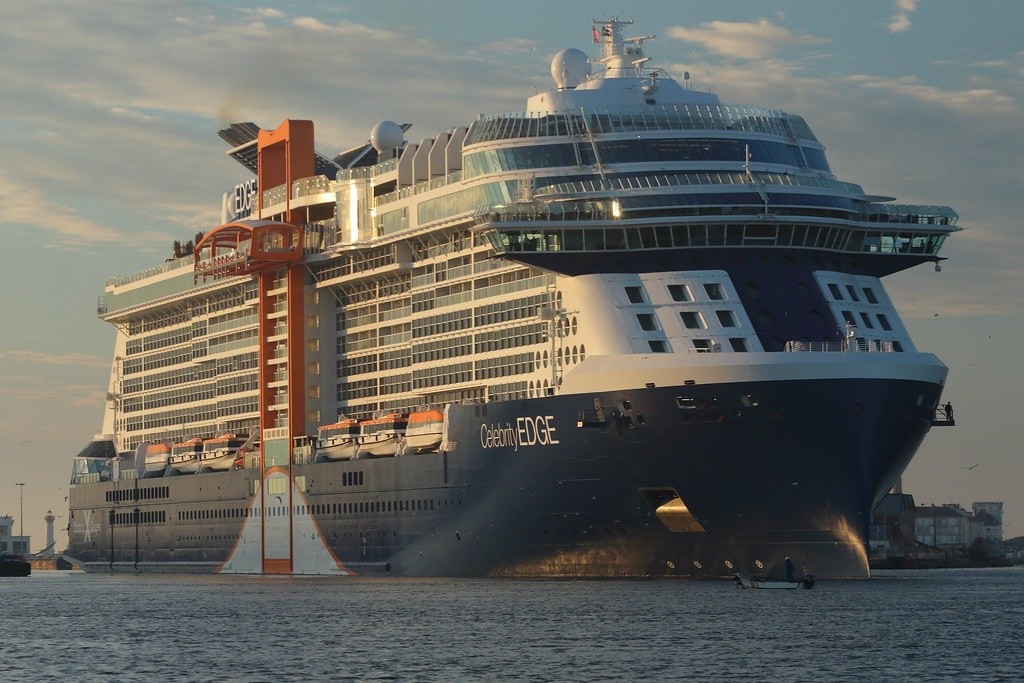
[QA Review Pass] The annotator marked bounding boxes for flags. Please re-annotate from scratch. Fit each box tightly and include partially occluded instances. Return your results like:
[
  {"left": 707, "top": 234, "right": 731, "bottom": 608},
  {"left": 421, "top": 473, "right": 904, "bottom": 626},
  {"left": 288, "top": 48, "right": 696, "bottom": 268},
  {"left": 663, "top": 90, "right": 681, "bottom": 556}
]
[
  {"left": 592, "top": 27, "right": 602, "bottom": 42},
  {"left": 601, "top": 27, "right": 611, "bottom": 37}
]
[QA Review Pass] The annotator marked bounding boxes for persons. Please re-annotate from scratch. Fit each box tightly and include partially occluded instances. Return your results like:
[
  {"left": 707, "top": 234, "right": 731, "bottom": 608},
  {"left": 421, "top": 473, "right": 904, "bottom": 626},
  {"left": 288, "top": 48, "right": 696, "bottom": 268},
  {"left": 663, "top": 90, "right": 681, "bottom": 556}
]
[
  {"left": 784, "top": 556, "right": 795, "bottom": 583},
  {"left": 920, "top": 237, "right": 934, "bottom": 254},
  {"left": 509, "top": 233, "right": 537, "bottom": 252},
  {"left": 944, "top": 401, "right": 953, "bottom": 422}
]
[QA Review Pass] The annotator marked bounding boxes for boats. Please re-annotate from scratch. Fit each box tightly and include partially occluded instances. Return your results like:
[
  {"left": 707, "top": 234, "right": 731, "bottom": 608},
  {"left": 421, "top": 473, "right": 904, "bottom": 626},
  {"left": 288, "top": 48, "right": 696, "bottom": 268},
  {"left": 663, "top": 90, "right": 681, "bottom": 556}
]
[
  {"left": 200, "top": 434, "right": 242, "bottom": 471},
  {"left": 356, "top": 413, "right": 408, "bottom": 456},
  {"left": 731, "top": 570, "right": 816, "bottom": 589},
  {"left": 145, "top": 444, "right": 173, "bottom": 474},
  {"left": 170, "top": 438, "right": 204, "bottom": 473},
  {"left": 0, "top": 554, "right": 32, "bottom": 577},
  {"left": 316, "top": 417, "right": 370, "bottom": 460},
  {"left": 405, "top": 410, "right": 444, "bottom": 449}
]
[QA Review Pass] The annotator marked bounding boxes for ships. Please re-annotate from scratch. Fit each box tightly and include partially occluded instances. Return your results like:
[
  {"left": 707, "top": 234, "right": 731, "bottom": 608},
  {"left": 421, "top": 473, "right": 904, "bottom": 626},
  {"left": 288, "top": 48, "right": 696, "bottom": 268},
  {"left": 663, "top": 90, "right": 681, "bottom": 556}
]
[{"left": 69, "top": 16, "right": 965, "bottom": 582}]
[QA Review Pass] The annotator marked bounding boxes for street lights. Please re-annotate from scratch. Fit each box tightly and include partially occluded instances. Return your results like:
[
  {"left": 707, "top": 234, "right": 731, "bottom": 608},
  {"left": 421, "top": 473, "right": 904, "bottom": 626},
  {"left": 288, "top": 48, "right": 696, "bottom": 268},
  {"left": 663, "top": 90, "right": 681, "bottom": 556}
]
[
  {"left": 921, "top": 503, "right": 926, "bottom": 544},
  {"left": 15, "top": 482, "right": 25, "bottom": 556},
  {"left": 932, "top": 502, "right": 936, "bottom": 548}
]
